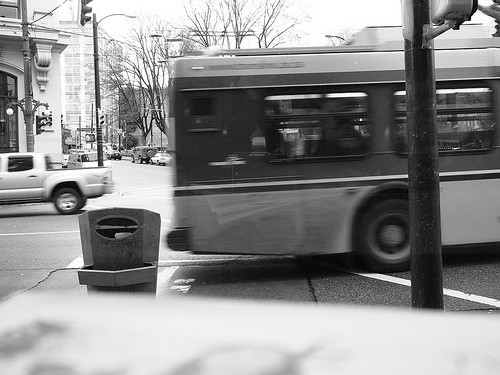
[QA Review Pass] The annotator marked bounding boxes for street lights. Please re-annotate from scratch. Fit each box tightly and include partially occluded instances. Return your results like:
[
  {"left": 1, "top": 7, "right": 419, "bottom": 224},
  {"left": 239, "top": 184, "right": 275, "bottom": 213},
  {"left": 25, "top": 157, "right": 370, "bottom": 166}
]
[{"left": 91, "top": 13, "right": 137, "bottom": 166}]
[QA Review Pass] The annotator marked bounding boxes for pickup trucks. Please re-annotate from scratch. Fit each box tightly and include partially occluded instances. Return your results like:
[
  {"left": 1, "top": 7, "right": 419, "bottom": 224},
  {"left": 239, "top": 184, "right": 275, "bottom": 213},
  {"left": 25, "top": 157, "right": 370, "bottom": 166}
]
[{"left": 1, "top": 153, "right": 113, "bottom": 215}]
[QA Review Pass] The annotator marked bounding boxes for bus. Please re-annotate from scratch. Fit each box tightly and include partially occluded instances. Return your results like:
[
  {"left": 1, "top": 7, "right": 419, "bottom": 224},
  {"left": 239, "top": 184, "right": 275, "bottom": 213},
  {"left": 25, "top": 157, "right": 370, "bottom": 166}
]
[{"left": 162, "top": 25, "right": 500, "bottom": 273}]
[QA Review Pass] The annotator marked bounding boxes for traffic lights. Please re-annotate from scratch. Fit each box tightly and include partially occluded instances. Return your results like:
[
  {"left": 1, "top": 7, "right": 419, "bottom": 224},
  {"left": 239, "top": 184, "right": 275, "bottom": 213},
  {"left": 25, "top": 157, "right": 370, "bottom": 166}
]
[
  {"left": 35, "top": 115, "right": 46, "bottom": 137},
  {"left": 76, "top": 0, "right": 92, "bottom": 33},
  {"left": 98, "top": 115, "right": 106, "bottom": 127}
]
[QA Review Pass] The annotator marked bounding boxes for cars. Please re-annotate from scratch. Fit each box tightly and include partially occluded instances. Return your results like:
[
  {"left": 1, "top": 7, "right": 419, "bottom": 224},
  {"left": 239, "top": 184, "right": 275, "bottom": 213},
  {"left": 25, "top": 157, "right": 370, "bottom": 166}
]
[{"left": 70, "top": 143, "right": 171, "bottom": 167}]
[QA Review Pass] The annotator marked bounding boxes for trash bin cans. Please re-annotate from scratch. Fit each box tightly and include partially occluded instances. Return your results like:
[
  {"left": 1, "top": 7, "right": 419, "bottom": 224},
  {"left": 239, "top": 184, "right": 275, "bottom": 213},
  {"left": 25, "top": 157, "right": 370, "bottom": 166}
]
[{"left": 76, "top": 208, "right": 161, "bottom": 299}]
